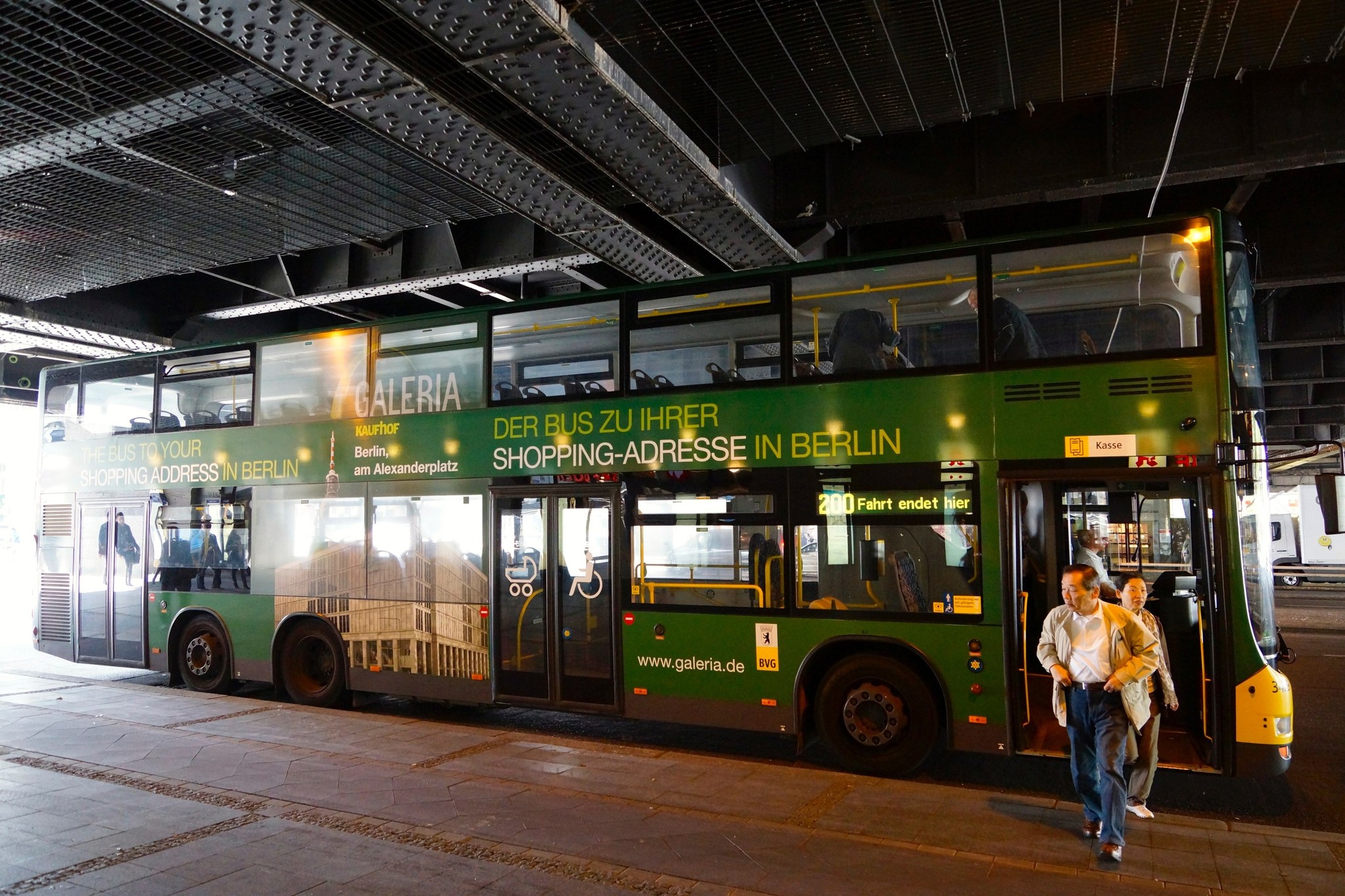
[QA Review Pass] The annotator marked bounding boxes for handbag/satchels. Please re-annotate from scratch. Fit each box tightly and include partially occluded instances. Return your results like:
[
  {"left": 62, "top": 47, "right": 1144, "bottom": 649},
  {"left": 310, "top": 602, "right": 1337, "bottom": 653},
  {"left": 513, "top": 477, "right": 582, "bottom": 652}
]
[
  {"left": 127, "top": 545, "right": 141, "bottom": 564},
  {"left": 244, "top": 566, "right": 251, "bottom": 577},
  {"left": 227, "top": 550, "right": 232, "bottom": 563}
]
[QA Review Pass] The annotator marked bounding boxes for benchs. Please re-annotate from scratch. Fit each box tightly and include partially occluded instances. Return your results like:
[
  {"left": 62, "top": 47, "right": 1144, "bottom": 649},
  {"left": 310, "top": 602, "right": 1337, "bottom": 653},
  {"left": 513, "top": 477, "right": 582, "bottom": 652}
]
[
  {"left": 113, "top": 402, "right": 332, "bottom": 434},
  {"left": 494, "top": 357, "right": 824, "bottom": 400}
]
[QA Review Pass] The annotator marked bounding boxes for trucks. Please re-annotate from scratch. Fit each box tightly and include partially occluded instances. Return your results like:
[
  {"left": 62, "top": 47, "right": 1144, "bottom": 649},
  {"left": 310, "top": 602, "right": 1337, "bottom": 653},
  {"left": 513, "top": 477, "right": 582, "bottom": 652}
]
[{"left": 1240, "top": 485, "right": 1345, "bottom": 586}]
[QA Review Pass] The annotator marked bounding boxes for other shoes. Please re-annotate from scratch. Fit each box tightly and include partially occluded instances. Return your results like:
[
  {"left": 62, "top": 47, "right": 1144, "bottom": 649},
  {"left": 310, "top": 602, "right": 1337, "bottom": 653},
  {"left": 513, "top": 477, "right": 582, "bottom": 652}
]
[
  {"left": 197, "top": 588, "right": 205, "bottom": 590},
  {"left": 212, "top": 587, "right": 225, "bottom": 590}
]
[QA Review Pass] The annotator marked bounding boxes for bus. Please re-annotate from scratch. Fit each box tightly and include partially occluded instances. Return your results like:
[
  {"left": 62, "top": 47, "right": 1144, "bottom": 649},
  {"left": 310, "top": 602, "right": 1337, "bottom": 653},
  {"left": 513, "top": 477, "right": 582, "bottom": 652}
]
[{"left": 26, "top": 199, "right": 1303, "bottom": 820}]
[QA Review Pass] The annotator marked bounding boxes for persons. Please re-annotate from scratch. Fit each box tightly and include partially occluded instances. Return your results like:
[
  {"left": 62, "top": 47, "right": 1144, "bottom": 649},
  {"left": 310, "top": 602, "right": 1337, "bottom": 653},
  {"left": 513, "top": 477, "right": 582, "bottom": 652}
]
[
  {"left": 965, "top": 286, "right": 1048, "bottom": 364},
  {"left": 153, "top": 522, "right": 249, "bottom": 593},
  {"left": 1181, "top": 534, "right": 1192, "bottom": 570},
  {"left": 1035, "top": 562, "right": 1160, "bottom": 862},
  {"left": 98, "top": 511, "right": 139, "bottom": 587},
  {"left": 1116, "top": 572, "right": 1179, "bottom": 818},
  {"left": 1074, "top": 529, "right": 1117, "bottom": 600},
  {"left": 826, "top": 308, "right": 900, "bottom": 374}
]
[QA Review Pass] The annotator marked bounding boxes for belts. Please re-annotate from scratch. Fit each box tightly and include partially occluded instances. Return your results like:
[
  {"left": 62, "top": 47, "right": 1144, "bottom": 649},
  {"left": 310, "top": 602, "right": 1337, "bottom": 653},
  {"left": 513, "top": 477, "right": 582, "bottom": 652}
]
[{"left": 1071, "top": 681, "right": 1107, "bottom": 690}]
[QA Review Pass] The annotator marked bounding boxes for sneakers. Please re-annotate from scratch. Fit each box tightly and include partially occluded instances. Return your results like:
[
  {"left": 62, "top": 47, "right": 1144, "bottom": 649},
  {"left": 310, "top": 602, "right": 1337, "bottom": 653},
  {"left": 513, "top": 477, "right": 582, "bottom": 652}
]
[{"left": 1126, "top": 804, "right": 1154, "bottom": 818}]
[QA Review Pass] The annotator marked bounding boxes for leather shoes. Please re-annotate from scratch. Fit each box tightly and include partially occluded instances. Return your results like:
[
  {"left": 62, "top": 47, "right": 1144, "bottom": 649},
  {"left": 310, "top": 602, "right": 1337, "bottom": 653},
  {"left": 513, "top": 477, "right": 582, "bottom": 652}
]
[
  {"left": 1100, "top": 843, "right": 1122, "bottom": 861},
  {"left": 1082, "top": 818, "right": 1102, "bottom": 839}
]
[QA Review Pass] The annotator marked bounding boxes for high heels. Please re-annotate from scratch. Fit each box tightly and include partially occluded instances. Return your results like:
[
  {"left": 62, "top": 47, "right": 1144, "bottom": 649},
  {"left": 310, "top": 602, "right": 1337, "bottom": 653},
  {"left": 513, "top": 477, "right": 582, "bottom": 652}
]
[
  {"left": 233, "top": 582, "right": 239, "bottom": 588},
  {"left": 243, "top": 582, "right": 250, "bottom": 589}
]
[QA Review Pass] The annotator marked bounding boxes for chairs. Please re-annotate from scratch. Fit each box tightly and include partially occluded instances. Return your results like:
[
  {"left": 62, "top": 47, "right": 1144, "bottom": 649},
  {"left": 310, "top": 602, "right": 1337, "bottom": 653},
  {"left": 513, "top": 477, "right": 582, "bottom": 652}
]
[{"left": 890, "top": 550, "right": 931, "bottom": 613}]
